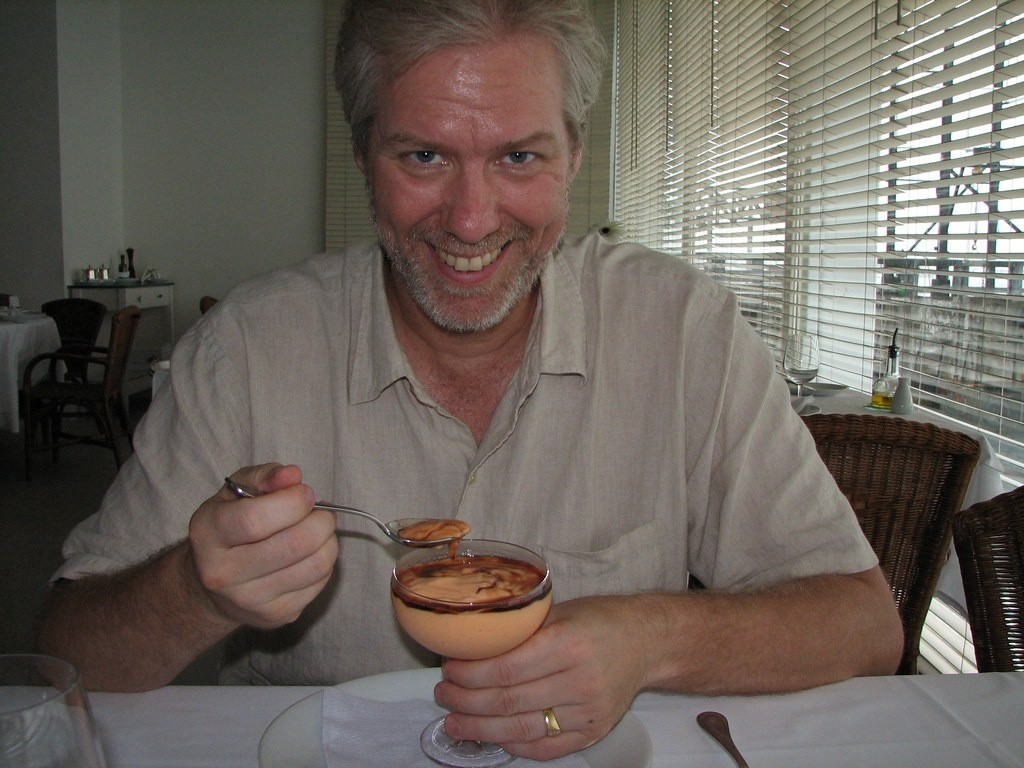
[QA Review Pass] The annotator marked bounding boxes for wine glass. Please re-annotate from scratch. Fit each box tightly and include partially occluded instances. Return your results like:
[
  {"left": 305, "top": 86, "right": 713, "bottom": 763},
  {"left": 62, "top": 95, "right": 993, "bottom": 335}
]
[
  {"left": 389, "top": 539, "right": 553, "bottom": 768},
  {"left": 782, "top": 334, "right": 822, "bottom": 415}
]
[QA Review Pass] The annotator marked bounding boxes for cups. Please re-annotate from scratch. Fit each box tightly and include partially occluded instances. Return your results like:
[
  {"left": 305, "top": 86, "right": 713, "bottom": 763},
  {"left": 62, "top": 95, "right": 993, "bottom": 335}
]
[{"left": 0, "top": 653, "right": 107, "bottom": 768}]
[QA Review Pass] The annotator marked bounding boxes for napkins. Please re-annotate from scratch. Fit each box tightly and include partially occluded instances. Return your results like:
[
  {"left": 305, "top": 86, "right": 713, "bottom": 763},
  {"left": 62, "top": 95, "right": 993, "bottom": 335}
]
[{"left": 320, "top": 684, "right": 590, "bottom": 768}]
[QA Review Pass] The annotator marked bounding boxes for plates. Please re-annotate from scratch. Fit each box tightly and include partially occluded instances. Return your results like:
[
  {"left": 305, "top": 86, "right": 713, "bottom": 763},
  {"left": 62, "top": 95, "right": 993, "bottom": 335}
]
[
  {"left": 257, "top": 668, "right": 652, "bottom": 768},
  {"left": 804, "top": 383, "right": 849, "bottom": 395}
]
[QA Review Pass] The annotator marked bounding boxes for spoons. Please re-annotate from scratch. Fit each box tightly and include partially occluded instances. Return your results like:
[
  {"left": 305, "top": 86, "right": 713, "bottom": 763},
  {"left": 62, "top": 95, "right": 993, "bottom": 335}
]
[{"left": 225, "top": 477, "right": 470, "bottom": 547}]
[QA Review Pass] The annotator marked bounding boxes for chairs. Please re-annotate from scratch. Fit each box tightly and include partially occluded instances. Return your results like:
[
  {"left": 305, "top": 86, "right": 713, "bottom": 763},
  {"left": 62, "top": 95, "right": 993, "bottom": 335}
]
[
  {"left": 800, "top": 414, "right": 1024, "bottom": 678},
  {"left": 18, "top": 298, "right": 144, "bottom": 480}
]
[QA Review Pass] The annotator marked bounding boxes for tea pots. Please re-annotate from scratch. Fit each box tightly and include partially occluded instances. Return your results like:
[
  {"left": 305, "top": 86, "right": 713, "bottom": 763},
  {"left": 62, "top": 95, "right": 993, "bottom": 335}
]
[{"left": 83, "top": 262, "right": 113, "bottom": 281}]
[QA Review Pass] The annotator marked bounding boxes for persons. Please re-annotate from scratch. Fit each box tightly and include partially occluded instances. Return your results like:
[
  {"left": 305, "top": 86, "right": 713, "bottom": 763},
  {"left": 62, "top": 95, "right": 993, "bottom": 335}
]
[{"left": 31, "top": 1, "right": 905, "bottom": 763}]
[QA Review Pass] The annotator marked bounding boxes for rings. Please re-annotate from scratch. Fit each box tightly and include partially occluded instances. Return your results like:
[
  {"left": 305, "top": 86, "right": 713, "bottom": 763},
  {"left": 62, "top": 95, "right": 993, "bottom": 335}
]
[{"left": 543, "top": 709, "right": 562, "bottom": 738}]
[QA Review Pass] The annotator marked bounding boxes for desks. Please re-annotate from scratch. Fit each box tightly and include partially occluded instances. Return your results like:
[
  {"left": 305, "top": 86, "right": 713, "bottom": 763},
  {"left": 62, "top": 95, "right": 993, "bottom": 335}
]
[
  {"left": 0, "top": 306, "right": 68, "bottom": 434},
  {"left": 67, "top": 279, "right": 173, "bottom": 397},
  {"left": 0, "top": 670, "right": 1024, "bottom": 768},
  {"left": 786, "top": 376, "right": 1008, "bottom": 627}
]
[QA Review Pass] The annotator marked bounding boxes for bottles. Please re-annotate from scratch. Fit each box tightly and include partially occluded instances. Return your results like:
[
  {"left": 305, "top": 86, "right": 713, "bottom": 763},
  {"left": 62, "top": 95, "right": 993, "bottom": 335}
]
[
  {"left": 870, "top": 346, "right": 901, "bottom": 408},
  {"left": 890, "top": 378, "right": 913, "bottom": 415}
]
